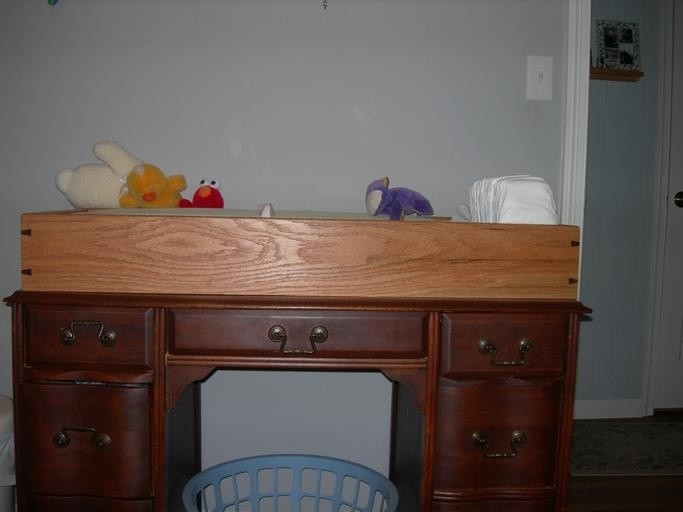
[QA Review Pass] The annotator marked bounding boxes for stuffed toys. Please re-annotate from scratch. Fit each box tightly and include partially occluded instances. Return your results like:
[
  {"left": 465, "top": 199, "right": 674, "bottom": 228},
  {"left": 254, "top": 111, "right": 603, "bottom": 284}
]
[
  {"left": 55, "top": 139, "right": 225, "bottom": 208},
  {"left": 365, "top": 177, "right": 434, "bottom": 220}
]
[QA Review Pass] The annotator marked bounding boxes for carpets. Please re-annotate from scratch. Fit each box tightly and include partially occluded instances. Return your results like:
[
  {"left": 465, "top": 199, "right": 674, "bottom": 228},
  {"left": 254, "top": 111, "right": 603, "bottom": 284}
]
[{"left": 568, "top": 416, "right": 683, "bottom": 477}]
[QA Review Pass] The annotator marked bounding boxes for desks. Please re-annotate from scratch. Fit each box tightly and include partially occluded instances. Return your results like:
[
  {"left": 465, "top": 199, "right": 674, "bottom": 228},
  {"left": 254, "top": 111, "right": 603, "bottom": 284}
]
[{"left": 2, "top": 289, "right": 595, "bottom": 510}]
